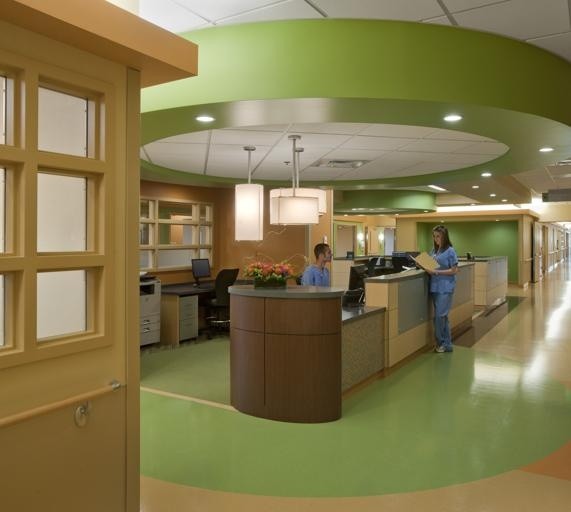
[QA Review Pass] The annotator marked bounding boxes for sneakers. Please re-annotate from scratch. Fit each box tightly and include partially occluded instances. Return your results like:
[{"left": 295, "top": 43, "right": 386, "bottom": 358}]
[{"left": 435, "top": 345, "right": 451, "bottom": 352}]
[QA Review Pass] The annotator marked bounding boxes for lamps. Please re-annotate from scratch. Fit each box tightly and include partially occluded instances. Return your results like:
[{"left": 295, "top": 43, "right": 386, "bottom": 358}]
[
  {"left": 271, "top": 134, "right": 320, "bottom": 226},
  {"left": 235, "top": 142, "right": 264, "bottom": 241},
  {"left": 271, "top": 136, "right": 326, "bottom": 218}
]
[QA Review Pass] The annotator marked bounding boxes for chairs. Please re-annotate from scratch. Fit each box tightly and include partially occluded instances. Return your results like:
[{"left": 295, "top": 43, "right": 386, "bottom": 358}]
[
  {"left": 204, "top": 268, "right": 240, "bottom": 341},
  {"left": 365, "top": 255, "right": 380, "bottom": 278}
]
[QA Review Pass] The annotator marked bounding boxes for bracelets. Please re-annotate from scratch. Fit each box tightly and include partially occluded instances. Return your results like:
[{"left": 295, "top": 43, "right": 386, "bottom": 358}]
[{"left": 435, "top": 271, "right": 439, "bottom": 276}]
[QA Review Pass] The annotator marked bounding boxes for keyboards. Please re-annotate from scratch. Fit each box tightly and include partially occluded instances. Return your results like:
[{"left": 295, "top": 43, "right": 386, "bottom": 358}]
[{"left": 199, "top": 284, "right": 211, "bottom": 288}]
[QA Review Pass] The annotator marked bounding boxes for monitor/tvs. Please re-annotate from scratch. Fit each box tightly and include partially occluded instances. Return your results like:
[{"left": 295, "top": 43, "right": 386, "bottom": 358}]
[
  {"left": 191, "top": 259, "right": 211, "bottom": 278},
  {"left": 349, "top": 264, "right": 366, "bottom": 290}
]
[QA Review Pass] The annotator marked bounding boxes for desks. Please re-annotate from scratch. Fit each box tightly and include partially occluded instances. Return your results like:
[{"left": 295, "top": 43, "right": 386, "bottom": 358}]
[{"left": 160, "top": 279, "right": 228, "bottom": 346}]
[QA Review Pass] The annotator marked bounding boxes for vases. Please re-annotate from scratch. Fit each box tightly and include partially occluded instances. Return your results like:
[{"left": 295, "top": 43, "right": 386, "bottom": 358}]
[{"left": 248, "top": 277, "right": 286, "bottom": 287}]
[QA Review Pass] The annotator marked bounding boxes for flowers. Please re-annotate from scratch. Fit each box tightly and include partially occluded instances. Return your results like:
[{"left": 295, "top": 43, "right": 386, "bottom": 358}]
[{"left": 249, "top": 259, "right": 296, "bottom": 279}]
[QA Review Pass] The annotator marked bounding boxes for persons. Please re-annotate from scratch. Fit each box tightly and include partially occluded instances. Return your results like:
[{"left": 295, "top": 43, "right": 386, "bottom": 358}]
[
  {"left": 301, "top": 243, "right": 333, "bottom": 287},
  {"left": 414, "top": 224, "right": 458, "bottom": 353}
]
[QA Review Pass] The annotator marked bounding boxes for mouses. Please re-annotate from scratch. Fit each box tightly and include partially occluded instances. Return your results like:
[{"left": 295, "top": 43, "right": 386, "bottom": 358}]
[{"left": 192, "top": 284, "right": 197, "bottom": 287}]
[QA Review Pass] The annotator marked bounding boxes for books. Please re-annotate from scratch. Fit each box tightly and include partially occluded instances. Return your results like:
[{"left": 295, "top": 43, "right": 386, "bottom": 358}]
[{"left": 409, "top": 252, "right": 440, "bottom": 270}]
